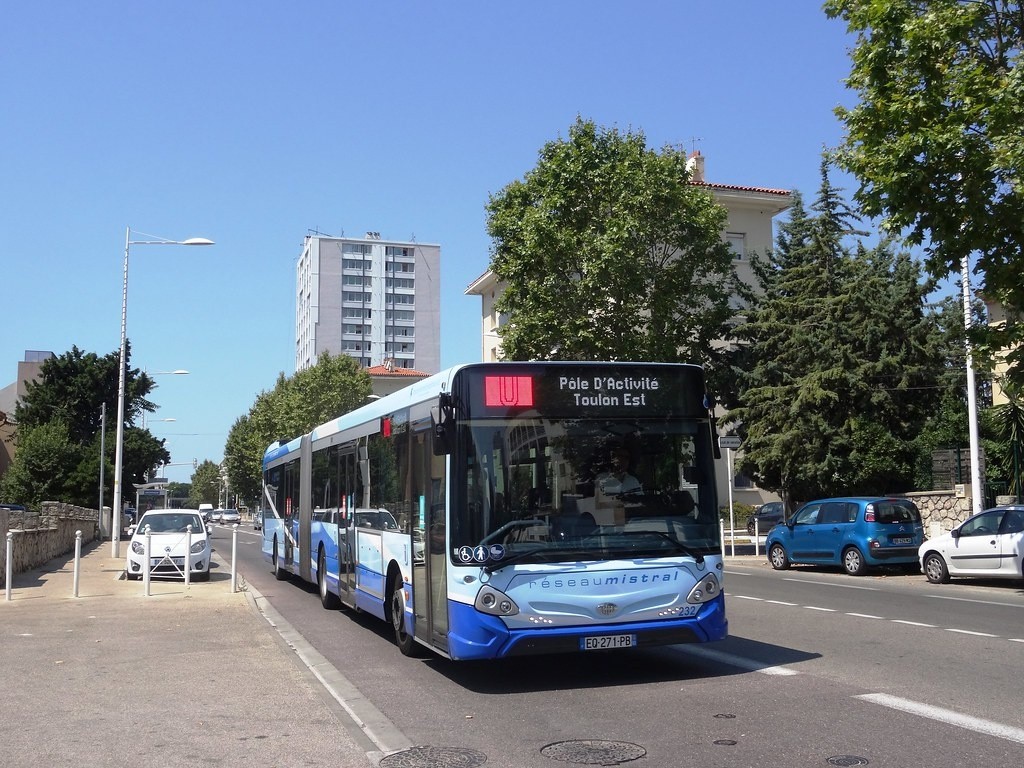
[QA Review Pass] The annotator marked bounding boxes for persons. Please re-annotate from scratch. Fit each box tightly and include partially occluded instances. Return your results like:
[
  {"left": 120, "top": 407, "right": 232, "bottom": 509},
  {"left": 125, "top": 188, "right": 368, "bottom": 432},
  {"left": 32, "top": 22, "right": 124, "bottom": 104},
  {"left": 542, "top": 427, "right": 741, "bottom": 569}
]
[{"left": 596, "top": 444, "right": 647, "bottom": 505}]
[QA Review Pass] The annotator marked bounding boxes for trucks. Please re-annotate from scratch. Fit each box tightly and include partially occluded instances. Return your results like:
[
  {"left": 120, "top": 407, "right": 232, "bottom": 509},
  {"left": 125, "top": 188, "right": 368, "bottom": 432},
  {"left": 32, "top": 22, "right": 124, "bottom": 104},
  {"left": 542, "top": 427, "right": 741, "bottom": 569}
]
[{"left": 199, "top": 504, "right": 213, "bottom": 517}]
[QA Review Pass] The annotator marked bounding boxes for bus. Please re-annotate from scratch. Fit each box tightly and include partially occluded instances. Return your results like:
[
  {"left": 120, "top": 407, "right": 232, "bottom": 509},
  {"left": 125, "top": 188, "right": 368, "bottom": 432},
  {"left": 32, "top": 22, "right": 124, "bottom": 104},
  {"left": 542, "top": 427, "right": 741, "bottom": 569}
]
[{"left": 260, "top": 361, "right": 730, "bottom": 659}]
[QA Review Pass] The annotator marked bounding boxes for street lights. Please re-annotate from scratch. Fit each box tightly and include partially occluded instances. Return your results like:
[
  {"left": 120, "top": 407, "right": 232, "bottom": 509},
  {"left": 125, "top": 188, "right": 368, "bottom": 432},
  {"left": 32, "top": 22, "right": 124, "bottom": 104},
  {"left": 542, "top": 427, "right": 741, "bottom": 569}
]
[
  {"left": 141, "top": 418, "right": 176, "bottom": 429},
  {"left": 211, "top": 481, "right": 222, "bottom": 508},
  {"left": 141, "top": 369, "right": 189, "bottom": 431},
  {"left": 161, "top": 458, "right": 197, "bottom": 478},
  {"left": 112, "top": 237, "right": 215, "bottom": 554}
]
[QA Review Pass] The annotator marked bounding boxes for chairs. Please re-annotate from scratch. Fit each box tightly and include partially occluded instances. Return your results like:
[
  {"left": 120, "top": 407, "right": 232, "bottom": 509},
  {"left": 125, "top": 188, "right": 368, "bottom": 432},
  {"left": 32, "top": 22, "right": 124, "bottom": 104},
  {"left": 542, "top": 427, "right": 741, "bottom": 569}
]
[
  {"left": 773, "top": 506, "right": 780, "bottom": 511},
  {"left": 179, "top": 516, "right": 197, "bottom": 532},
  {"left": 151, "top": 517, "right": 167, "bottom": 532},
  {"left": 824, "top": 505, "right": 844, "bottom": 522}
]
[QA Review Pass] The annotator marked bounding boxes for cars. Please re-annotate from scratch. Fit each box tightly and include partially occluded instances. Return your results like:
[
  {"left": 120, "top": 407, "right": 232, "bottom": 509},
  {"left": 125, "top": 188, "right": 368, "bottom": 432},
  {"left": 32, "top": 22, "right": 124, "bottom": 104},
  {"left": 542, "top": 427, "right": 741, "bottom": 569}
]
[
  {"left": 253, "top": 511, "right": 263, "bottom": 531},
  {"left": 918, "top": 504, "right": 1024, "bottom": 584},
  {"left": 311, "top": 508, "right": 428, "bottom": 568},
  {"left": 219, "top": 509, "right": 241, "bottom": 524},
  {"left": 766, "top": 497, "right": 924, "bottom": 575},
  {"left": 747, "top": 502, "right": 784, "bottom": 536},
  {"left": 210, "top": 509, "right": 224, "bottom": 522},
  {"left": 126, "top": 509, "right": 212, "bottom": 580}
]
